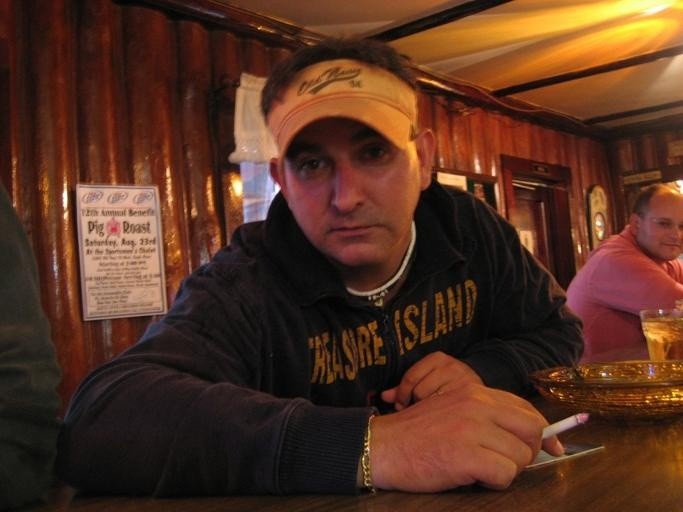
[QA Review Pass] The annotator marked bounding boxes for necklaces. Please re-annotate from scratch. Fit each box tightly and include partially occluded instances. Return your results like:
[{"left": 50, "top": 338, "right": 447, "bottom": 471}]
[{"left": 343, "top": 217, "right": 416, "bottom": 301}]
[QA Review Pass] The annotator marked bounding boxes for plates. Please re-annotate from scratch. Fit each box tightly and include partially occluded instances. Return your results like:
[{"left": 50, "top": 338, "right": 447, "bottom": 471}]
[{"left": 528, "top": 360, "right": 683, "bottom": 420}]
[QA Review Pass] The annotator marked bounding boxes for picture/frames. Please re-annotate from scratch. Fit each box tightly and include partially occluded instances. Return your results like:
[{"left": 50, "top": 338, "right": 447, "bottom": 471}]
[{"left": 74, "top": 184, "right": 169, "bottom": 321}]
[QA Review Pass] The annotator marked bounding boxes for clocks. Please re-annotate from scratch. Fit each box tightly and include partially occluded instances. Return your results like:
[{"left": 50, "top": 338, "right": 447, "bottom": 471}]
[{"left": 588, "top": 186, "right": 609, "bottom": 249}]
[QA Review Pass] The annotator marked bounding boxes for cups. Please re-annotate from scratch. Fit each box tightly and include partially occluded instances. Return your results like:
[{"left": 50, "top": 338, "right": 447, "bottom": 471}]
[{"left": 640, "top": 307, "right": 683, "bottom": 361}]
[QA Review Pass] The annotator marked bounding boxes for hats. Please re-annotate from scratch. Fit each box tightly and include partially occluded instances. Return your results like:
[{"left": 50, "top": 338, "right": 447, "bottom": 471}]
[{"left": 266, "top": 59, "right": 416, "bottom": 178}]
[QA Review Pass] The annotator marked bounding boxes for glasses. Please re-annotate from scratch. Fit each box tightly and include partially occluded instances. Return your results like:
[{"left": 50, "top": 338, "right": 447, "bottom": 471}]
[{"left": 638, "top": 212, "right": 683, "bottom": 232}]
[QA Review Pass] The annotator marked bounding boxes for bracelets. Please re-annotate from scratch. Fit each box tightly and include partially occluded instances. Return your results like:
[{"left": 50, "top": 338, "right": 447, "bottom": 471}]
[{"left": 359, "top": 410, "right": 376, "bottom": 489}]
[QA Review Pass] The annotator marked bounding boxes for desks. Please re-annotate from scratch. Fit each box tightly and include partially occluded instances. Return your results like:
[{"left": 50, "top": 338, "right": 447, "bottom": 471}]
[{"left": 18, "top": 402, "right": 683, "bottom": 512}]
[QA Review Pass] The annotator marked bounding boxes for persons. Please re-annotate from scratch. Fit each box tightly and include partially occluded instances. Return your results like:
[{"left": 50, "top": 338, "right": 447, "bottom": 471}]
[
  {"left": 0, "top": 184, "right": 62, "bottom": 511},
  {"left": 563, "top": 181, "right": 683, "bottom": 365},
  {"left": 52, "top": 32, "right": 584, "bottom": 501}
]
